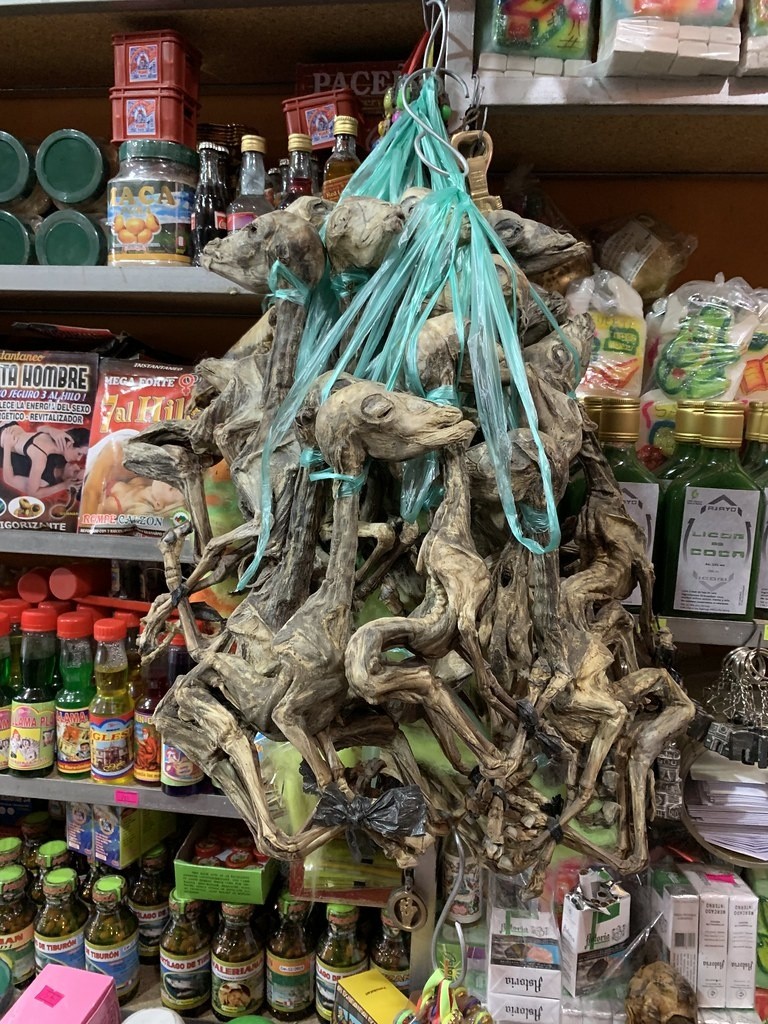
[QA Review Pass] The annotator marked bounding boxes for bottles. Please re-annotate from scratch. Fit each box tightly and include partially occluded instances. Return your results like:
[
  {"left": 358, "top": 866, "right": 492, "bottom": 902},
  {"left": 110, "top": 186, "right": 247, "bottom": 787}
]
[
  {"left": 233, "top": 133, "right": 272, "bottom": 233},
  {"left": 106, "top": 137, "right": 193, "bottom": 266},
  {"left": 322, "top": 113, "right": 361, "bottom": 200},
  {"left": 282, "top": 133, "right": 318, "bottom": 206},
  {"left": 0, "top": 803, "right": 488, "bottom": 1024},
  {"left": 0, "top": 559, "right": 229, "bottom": 798},
  {"left": 0, "top": 129, "right": 111, "bottom": 266},
  {"left": 581, "top": 396, "right": 768, "bottom": 621},
  {"left": 190, "top": 138, "right": 230, "bottom": 253}
]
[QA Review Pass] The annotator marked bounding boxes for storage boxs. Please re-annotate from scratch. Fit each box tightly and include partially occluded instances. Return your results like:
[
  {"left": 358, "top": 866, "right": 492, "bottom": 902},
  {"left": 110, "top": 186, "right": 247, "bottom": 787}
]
[
  {"left": 0, "top": 963, "right": 123, "bottom": 1024},
  {"left": 281, "top": 87, "right": 369, "bottom": 157},
  {"left": 108, "top": 87, "right": 203, "bottom": 152},
  {"left": 173, "top": 816, "right": 280, "bottom": 905},
  {"left": 93, "top": 803, "right": 140, "bottom": 870},
  {"left": 487, "top": 862, "right": 763, "bottom": 1024},
  {"left": 140, "top": 809, "right": 161, "bottom": 855},
  {"left": 331, "top": 969, "right": 416, "bottom": 1024},
  {"left": 107, "top": 28, "right": 206, "bottom": 99},
  {"left": 65, "top": 800, "right": 95, "bottom": 859},
  {"left": 160, "top": 811, "right": 176, "bottom": 840}
]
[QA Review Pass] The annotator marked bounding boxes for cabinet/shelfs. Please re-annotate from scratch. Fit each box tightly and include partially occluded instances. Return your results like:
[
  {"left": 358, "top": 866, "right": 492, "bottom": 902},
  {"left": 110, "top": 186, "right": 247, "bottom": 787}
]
[{"left": 0, "top": 0, "right": 768, "bottom": 989}]
